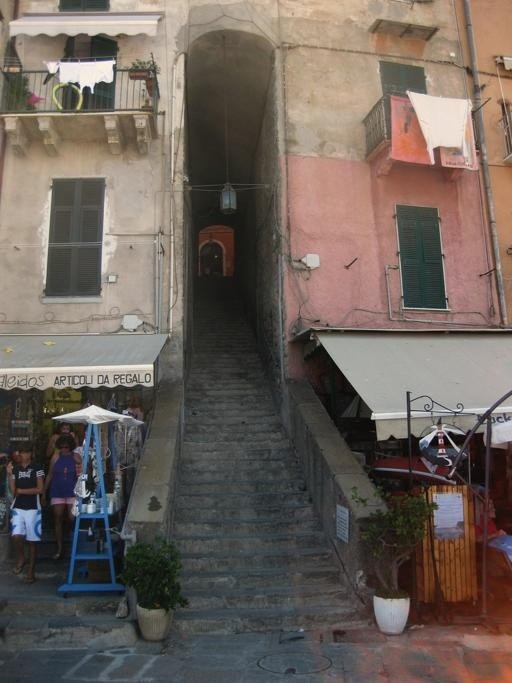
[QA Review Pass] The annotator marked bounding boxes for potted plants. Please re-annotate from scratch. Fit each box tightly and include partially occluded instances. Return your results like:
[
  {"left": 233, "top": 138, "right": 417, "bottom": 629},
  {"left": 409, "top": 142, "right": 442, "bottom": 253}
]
[
  {"left": 349, "top": 467, "right": 440, "bottom": 635},
  {"left": 119, "top": 532, "right": 193, "bottom": 642},
  {"left": 7, "top": 73, "right": 33, "bottom": 109},
  {"left": 129, "top": 58, "right": 161, "bottom": 80}
]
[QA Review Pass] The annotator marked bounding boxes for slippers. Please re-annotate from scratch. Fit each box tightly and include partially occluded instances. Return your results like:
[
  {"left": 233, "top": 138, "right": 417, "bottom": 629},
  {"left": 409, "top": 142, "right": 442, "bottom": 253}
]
[
  {"left": 13, "top": 561, "right": 25, "bottom": 574},
  {"left": 24, "top": 574, "right": 35, "bottom": 583}
]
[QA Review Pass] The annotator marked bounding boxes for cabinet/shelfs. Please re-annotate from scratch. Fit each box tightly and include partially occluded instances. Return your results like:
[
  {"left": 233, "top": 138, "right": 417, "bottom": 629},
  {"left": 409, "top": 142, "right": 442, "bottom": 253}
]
[{"left": 52, "top": 404, "right": 145, "bottom": 596}]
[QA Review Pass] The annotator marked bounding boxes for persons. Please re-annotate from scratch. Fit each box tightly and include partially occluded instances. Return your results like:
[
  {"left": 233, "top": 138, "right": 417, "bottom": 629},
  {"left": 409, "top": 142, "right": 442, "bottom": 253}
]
[
  {"left": 5, "top": 421, "right": 82, "bottom": 583},
  {"left": 476, "top": 500, "right": 512, "bottom": 563}
]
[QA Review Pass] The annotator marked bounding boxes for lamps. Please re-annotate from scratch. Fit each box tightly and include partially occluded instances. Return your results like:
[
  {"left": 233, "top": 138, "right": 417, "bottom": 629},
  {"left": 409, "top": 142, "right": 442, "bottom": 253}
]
[{"left": 220, "top": 103, "right": 237, "bottom": 212}]
